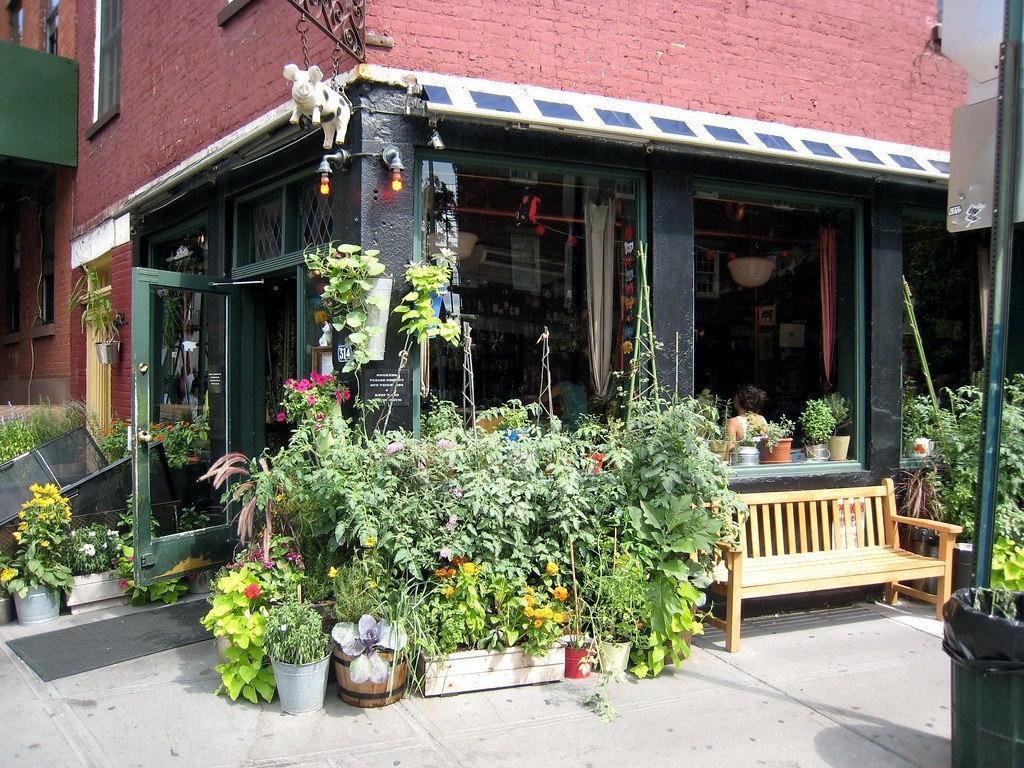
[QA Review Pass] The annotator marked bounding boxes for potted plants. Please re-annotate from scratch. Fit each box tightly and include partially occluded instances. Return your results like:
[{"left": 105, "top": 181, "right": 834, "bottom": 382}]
[
  {"left": 709, "top": 394, "right": 735, "bottom": 461},
  {"left": 897, "top": 374, "right": 936, "bottom": 459},
  {"left": 822, "top": 392, "right": 854, "bottom": 461},
  {"left": 755, "top": 414, "right": 796, "bottom": 464},
  {"left": 65, "top": 257, "right": 123, "bottom": 365},
  {"left": 889, "top": 455, "right": 945, "bottom": 603},
  {"left": 565, "top": 505, "right": 654, "bottom": 724},
  {"left": 796, "top": 398, "right": 836, "bottom": 463}
]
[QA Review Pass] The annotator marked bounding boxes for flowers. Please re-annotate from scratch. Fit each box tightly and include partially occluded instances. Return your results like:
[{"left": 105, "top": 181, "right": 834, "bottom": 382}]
[
  {"left": 325, "top": 534, "right": 390, "bottom": 626},
  {"left": 65, "top": 521, "right": 125, "bottom": 577},
  {"left": 0, "top": 482, "right": 77, "bottom": 601},
  {"left": 0, "top": 550, "right": 26, "bottom": 592},
  {"left": 261, "top": 595, "right": 332, "bottom": 666},
  {"left": 420, "top": 556, "right": 569, "bottom": 660}
]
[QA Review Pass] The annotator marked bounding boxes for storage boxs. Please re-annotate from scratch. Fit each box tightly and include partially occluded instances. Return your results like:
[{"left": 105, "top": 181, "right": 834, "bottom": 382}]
[
  {"left": 476, "top": 415, "right": 505, "bottom": 433},
  {"left": 778, "top": 322, "right": 806, "bottom": 348},
  {"left": 934, "top": 319, "right": 963, "bottom": 340}
]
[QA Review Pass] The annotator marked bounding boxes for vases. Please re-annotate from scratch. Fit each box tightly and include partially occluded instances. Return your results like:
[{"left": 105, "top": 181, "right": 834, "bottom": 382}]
[
  {"left": 13, "top": 584, "right": 62, "bottom": 626},
  {"left": 329, "top": 637, "right": 409, "bottom": 709},
  {"left": 0, "top": 590, "right": 16, "bottom": 627},
  {"left": 65, "top": 570, "right": 134, "bottom": 615},
  {"left": 186, "top": 569, "right": 213, "bottom": 594},
  {"left": 269, "top": 642, "right": 336, "bottom": 716},
  {"left": 414, "top": 636, "right": 566, "bottom": 700}
]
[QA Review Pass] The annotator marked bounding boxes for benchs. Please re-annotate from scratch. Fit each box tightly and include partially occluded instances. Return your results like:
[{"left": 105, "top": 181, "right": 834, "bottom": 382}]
[{"left": 685, "top": 477, "right": 963, "bottom": 654}]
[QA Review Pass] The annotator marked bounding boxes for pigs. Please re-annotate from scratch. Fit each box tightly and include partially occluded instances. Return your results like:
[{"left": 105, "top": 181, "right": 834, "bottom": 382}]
[{"left": 283, "top": 63, "right": 354, "bottom": 150}]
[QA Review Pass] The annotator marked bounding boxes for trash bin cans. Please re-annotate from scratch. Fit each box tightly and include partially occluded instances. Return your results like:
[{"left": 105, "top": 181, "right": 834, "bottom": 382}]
[{"left": 949, "top": 588, "right": 1024, "bottom": 768}]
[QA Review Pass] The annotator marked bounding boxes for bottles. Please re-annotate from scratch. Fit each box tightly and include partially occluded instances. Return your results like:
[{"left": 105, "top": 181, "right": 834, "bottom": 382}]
[{"left": 420, "top": 289, "right": 575, "bottom": 407}]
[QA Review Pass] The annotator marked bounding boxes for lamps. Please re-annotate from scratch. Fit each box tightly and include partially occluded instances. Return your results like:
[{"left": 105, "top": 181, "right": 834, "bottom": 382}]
[
  {"left": 314, "top": 149, "right": 353, "bottom": 194},
  {"left": 427, "top": 211, "right": 478, "bottom": 262},
  {"left": 382, "top": 145, "right": 407, "bottom": 192},
  {"left": 725, "top": 241, "right": 778, "bottom": 288},
  {"left": 426, "top": 117, "right": 446, "bottom": 149}
]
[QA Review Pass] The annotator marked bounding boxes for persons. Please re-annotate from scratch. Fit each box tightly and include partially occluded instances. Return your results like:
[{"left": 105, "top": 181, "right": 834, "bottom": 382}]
[
  {"left": 542, "top": 363, "right": 587, "bottom": 430},
  {"left": 181, "top": 365, "right": 194, "bottom": 393},
  {"left": 724, "top": 385, "right": 768, "bottom": 460},
  {"left": 182, "top": 378, "right": 198, "bottom": 418}
]
